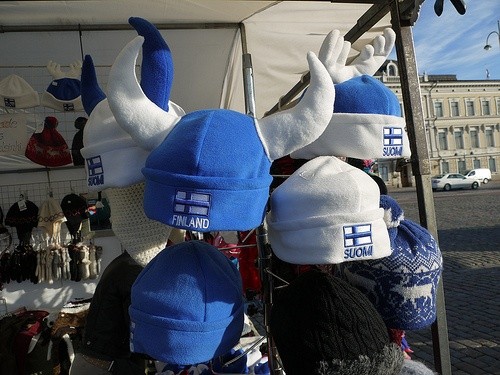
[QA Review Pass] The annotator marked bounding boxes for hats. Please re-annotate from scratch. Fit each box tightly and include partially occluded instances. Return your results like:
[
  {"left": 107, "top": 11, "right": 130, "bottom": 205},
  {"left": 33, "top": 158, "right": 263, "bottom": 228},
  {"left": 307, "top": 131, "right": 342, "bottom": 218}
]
[
  {"left": 81, "top": 240, "right": 245, "bottom": 366},
  {"left": 83, "top": 16, "right": 335, "bottom": 233},
  {"left": 41, "top": 60, "right": 82, "bottom": 111},
  {"left": 271, "top": 194, "right": 443, "bottom": 375},
  {"left": 25, "top": 117, "right": 88, "bottom": 166},
  {"left": 1, "top": 192, "right": 86, "bottom": 244},
  {"left": 266, "top": 28, "right": 412, "bottom": 263},
  {"left": 0, "top": 74, "right": 40, "bottom": 109}
]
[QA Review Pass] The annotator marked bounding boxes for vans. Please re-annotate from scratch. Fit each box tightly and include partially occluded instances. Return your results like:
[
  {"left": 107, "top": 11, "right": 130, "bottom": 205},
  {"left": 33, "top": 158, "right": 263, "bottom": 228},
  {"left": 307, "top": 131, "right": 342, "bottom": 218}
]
[{"left": 460, "top": 168, "right": 492, "bottom": 184}]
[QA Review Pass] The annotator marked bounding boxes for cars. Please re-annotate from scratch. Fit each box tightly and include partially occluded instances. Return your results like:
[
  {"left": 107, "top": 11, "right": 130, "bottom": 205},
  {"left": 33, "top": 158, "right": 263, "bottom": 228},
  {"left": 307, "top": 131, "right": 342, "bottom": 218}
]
[{"left": 431, "top": 172, "right": 480, "bottom": 191}]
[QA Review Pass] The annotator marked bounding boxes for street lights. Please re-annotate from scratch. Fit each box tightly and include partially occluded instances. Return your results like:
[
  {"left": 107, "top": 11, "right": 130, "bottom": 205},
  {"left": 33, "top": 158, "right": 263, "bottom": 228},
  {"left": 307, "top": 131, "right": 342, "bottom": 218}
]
[{"left": 483, "top": 20, "right": 500, "bottom": 52}]
